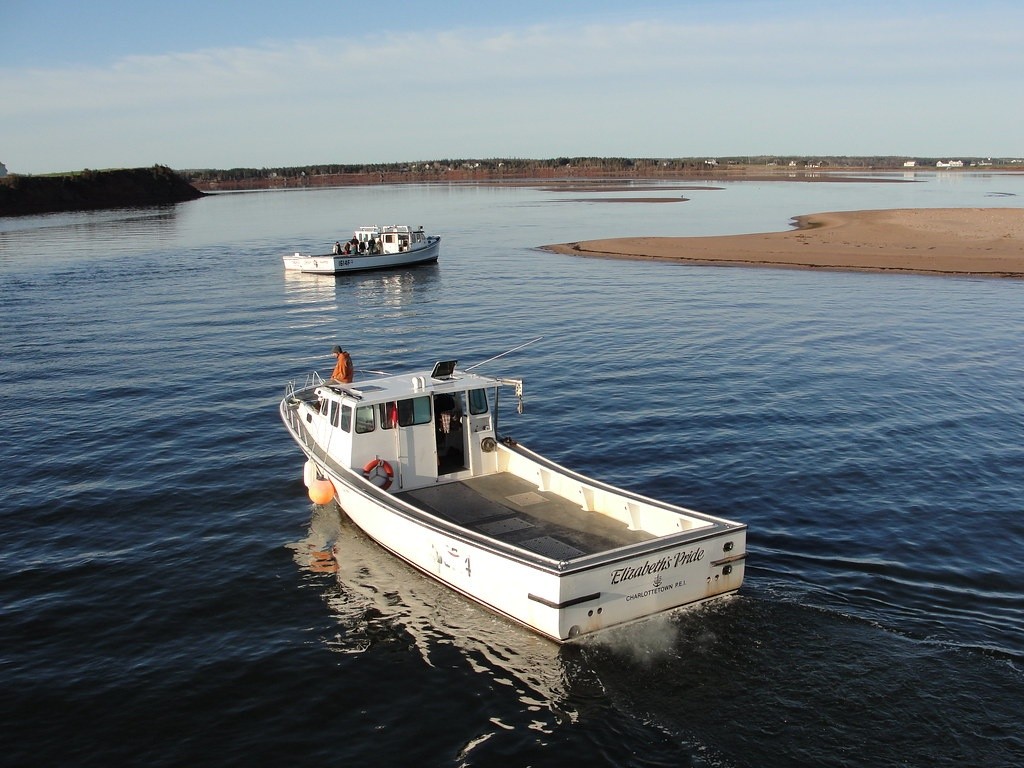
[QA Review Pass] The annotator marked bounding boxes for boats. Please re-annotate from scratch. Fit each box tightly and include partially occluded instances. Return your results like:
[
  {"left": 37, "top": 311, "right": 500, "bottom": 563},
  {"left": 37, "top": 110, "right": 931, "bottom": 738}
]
[
  {"left": 281, "top": 335, "right": 746, "bottom": 643},
  {"left": 283, "top": 224, "right": 441, "bottom": 276}
]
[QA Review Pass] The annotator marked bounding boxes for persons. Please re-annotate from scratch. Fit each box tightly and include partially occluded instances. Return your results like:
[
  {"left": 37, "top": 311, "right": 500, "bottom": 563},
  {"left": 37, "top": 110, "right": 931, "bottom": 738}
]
[
  {"left": 376, "top": 239, "right": 382, "bottom": 254},
  {"left": 330, "top": 346, "right": 354, "bottom": 383},
  {"left": 332, "top": 241, "right": 342, "bottom": 255},
  {"left": 351, "top": 236, "right": 359, "bottom": 251},
  {"left": 343, "top": 242, "right": 350, "bottom": 254},
  {"left": 359, "top": 242, "right": 365, "bottom": 252},
  {"left": 368, "top": 237, "right": 375, "bottom": 252}
]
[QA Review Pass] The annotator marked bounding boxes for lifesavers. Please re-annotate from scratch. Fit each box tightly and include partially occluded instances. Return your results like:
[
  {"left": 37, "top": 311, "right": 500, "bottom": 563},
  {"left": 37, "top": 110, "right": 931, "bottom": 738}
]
[{"left": 362, "top": 458, "right": 393, "bottom": 492}]
